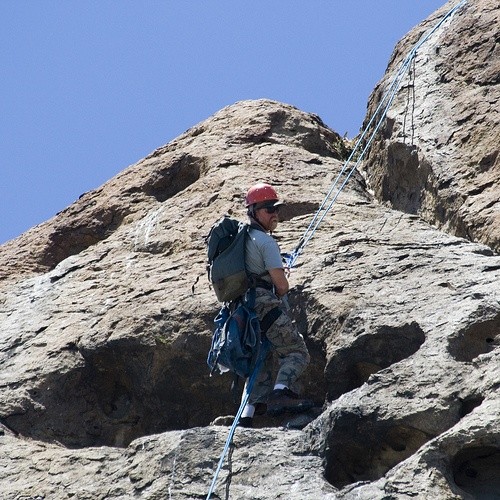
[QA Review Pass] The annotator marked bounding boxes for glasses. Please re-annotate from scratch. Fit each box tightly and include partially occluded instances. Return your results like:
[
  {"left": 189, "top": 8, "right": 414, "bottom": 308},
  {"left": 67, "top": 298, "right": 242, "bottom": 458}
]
[{"left": 255, "top": 206, "right": 279, "bottom": 214}]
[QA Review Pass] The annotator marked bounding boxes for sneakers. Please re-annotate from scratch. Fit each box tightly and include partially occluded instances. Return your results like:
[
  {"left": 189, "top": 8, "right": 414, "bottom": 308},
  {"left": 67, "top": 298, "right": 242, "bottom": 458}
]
[
  {"left": 232, "top": 416, "right": 253, "bottom": 428},
  {"left": 267, "top": 387, "right": 314, "bottom": 417}
]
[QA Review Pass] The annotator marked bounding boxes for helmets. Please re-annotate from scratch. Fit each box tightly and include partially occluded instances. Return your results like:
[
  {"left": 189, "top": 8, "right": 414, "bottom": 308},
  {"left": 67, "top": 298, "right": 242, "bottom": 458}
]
[{"left": 245, "top": 183, "right": 279, "bottom": 208}]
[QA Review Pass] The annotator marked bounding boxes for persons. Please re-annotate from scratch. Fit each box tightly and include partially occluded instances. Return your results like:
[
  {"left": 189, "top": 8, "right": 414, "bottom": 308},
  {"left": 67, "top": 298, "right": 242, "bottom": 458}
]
[{"left": 207, "top": 182, "right": 314, "bottom": 427}]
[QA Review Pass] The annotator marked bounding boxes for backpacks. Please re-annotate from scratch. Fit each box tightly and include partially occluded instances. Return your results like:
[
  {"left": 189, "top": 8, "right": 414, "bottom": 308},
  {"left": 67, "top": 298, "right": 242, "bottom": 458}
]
[{"left": 206, "top": 216, "right": 266, "bottom": 302}]
